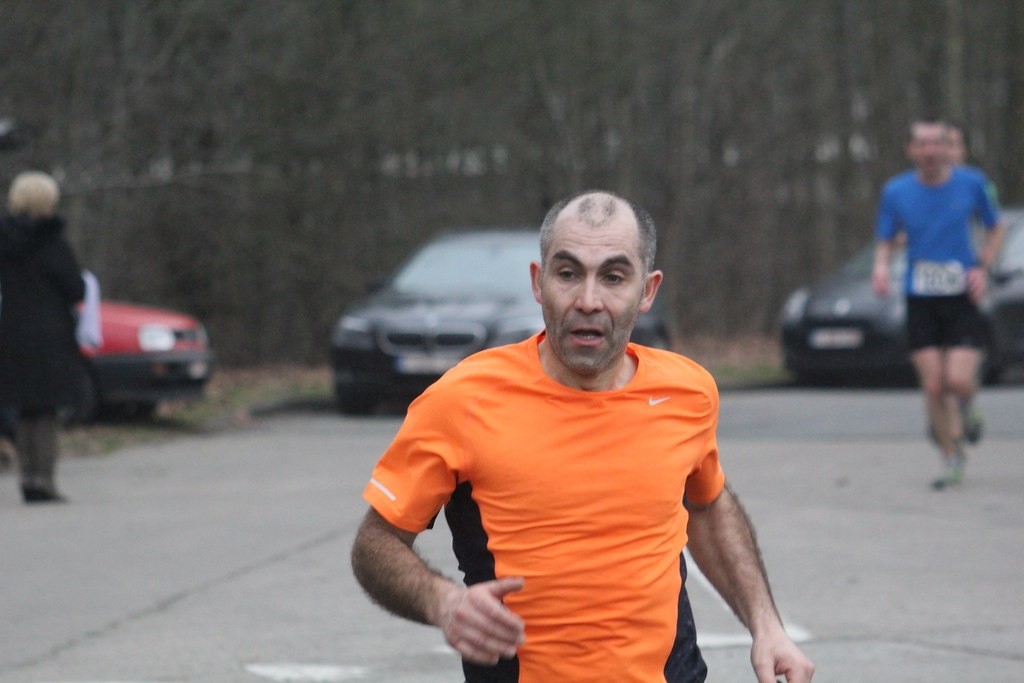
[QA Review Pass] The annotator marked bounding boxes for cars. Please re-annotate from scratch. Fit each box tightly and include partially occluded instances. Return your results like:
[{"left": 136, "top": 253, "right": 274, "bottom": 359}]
[
  {"left": 773, "top": 202, "right": 1024, "bottom": 389},
  {"left": 327, "top": 227, "right": 680, "bottom": 409},
  {"left": 54, "top": 296, "right": 212, "bottom": 433}
]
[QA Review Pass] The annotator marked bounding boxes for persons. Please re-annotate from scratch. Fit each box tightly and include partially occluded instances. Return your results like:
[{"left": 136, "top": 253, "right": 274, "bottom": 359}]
[
  {"left": 869, "top": 115, "right": 1004, "bottom": 490},
  {"left": 349, "top": 190, "right": 816, "bottom": 683},
  {"left": 0, "top": 171, "right": 88, "bottom": 504}
]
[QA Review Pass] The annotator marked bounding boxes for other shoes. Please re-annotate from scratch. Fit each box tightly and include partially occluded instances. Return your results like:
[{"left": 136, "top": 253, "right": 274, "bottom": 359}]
[
  {"left": 935, "top": 462, "right": 963, "bottom": 487},
  {"left": 963, "top": 403, "right": 984, "bottom": 443},
  {"left": 23, "top": 486, "right": 65, "bottom": 502}
]
